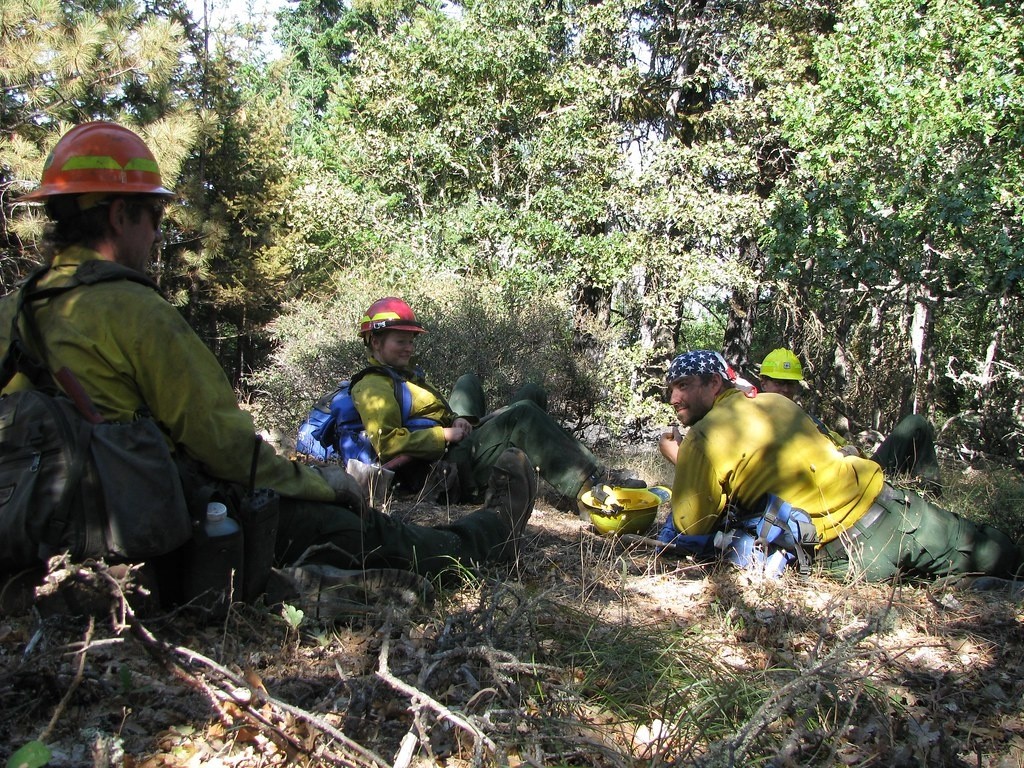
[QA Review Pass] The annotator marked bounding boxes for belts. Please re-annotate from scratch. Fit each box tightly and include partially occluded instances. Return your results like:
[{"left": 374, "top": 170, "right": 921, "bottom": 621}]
[{"left": 816, "top": 483, "right": 891, "bottom": 559}]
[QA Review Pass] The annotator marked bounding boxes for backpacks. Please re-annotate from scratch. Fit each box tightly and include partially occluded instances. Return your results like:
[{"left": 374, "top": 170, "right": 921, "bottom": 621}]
[
  {"left": 296, "top": 365, "right": 446, "bottom": 470},
  {"left": 0, "top": 259, "right": 193, "bottom": 569}
]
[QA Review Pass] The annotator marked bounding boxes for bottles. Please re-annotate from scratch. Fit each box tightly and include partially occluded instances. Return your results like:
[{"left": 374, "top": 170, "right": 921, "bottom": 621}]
[
  {"left": 714, "top": 529, "right": 736, "bottom": 554},
  {"left": 204, "top": 502, "right": 240, "bottom": 538}
]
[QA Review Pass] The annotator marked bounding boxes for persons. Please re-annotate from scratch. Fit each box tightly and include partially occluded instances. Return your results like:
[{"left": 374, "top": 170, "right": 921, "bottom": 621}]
[
  {"left": 330, "top": 298, "right": 646, "bottom": 519},
  {"left": 758, "top": 348, "right": 942, "bottom": 497},
  {"left": 654, "top": 348, "right": 1024, "bottom": 582},
  {"left": 0, "top": 122, "right": 540, "bottom": 614}
]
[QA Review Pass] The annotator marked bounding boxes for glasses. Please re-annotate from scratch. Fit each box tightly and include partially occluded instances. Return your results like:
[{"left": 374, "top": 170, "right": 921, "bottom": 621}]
[{"left": 101, "top": 200, "right": 163, "bottom": 234}]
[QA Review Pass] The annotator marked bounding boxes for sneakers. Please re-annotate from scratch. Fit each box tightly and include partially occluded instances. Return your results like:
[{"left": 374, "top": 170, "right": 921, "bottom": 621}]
[
  {"left": 484, "top": 447, "right": 536, "bottom": 563},
  {"left": 266, "top": 564, "right": 434, "bottom": 630}
]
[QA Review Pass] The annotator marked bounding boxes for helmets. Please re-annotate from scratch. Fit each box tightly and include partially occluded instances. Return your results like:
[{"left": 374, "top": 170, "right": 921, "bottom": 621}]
[
  {"left": 580, "top": 482, "right": 660, "bottom": 536},
  {"left": 15, "top": 121, "right": 177, "bottom": 200},
  {"left": 358, "top": 297, "right": 428, "bottom": 337},
  {"left": 759, "top": 347, "right": 805, "bottom": 380}
]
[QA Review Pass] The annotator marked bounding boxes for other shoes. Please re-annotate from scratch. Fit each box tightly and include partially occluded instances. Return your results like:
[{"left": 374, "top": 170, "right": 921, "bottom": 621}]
[
  {"left": 598, "top": 469, "right": 646, "bottom": 488},
  {"left": 969, "top": 576, "right": 1024, "bottom": 602}
]
